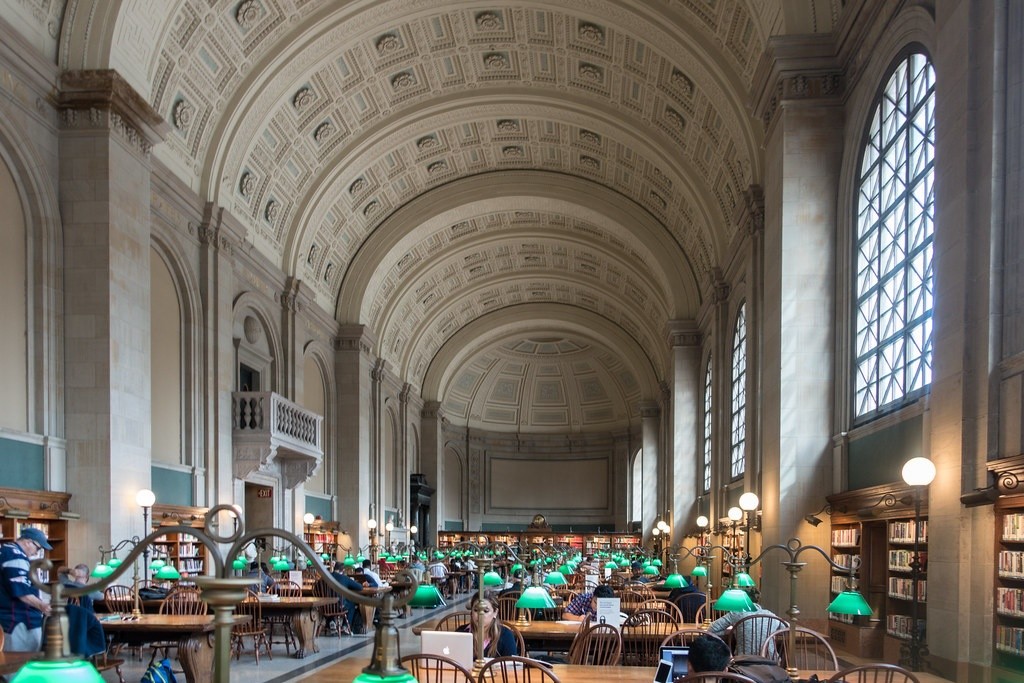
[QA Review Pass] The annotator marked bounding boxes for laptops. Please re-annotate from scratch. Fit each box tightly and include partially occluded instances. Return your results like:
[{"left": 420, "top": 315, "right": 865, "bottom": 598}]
[
  {"left": 421, "top": 630, "right": 474, "bottom": 671},
  {"left": 659, "top": 646, "right": 690, "bottom": 683}
]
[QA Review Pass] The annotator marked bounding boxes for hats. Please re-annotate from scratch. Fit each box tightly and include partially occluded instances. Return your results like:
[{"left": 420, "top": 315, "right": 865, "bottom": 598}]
[
  {"left": 632, "top": 562, "right": 642, "bottom": 568},
  {"left": 21, "top": 529, "right": 53, "bottom": 550}
]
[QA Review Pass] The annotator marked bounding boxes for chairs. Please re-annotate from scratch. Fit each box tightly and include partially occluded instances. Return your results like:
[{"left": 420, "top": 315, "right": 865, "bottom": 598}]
[{"left": 85, "top": 586, "right": 921, "bottom": 683}]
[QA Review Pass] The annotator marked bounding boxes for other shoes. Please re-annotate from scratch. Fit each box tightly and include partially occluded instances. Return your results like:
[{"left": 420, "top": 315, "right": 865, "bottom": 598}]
[
  {"left": 341, "top": 626, "right": 350, "bottom": 635},
  {"left": 329, "top": 621, "right": 338, "bottom": 635}
]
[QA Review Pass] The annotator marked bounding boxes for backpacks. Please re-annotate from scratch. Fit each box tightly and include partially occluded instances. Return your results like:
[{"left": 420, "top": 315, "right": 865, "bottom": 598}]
[{"left": 140, "top": 659, "right": 178, "bottom": 683}]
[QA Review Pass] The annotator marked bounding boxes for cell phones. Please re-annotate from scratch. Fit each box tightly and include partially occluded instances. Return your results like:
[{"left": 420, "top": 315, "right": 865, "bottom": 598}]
[{"left": 653, "top": 659, "right": 673, "bottom": 683}]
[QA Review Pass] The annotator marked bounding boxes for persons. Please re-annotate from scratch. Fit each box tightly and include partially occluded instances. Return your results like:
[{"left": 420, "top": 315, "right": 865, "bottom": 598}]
[
  {"left": 498, "top": 569, "right": 543, "bottom": 621},
  {"left": 450, "top": 556, "right": 478, "bottom": 588},
  {"left": 355, "top": 560, "right": 392, "bottom": 588},
  {"left": 429, "top": 562, "right": 449, "bottom": 585},
  {"left": 249, "top": 562, "right": 280, "bottom": 593},
  {"left": 315, "top": 562, "right": 363, "bottom": 634},
  {"left": 409, "top": 556, "right": 425, "bottom": 573},
  {"left": 57, "top": 564, "right": 104, "bottom": 606},
  {"left": 581, "top": 557, "right": 594, "bottom": 565},
  {"left": 0, "top": 528, "right": 53, "bottom": 652},
  {"left": 625, "top": 562, "right": 651, "bottom": 584},
  {"left": 687, "top": 635, "right": 730, "bottom": 683},
  {"left": 562, "top": 586, "right": 615, "bottom": 622},
  {"left": 709, "top": 588, "right": 790, "bottom": 661},
  {"left": 666, "top": 575, "right": 706, "bottom": 623},
  {"left": 525, "top": 560, "right": 550, "bottom": 590},
  {"left": 455, "top": 590, "right": 518, "bottom": 662}
]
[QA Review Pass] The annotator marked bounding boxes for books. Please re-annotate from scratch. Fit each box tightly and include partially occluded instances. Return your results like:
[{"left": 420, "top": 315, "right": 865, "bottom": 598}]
[
  {"left": 439, "top": 534, "right": 640, "bottom": 555},
  {"left": 996, "top": 513, "right": 1024, "bottom": 656},
  {"left": 17, "top": 522, "right": 50, "bottom": 583},
  {"left": 153, "top": 528, "right": 203, "bottom": 589},
  {"left": 304, "top": 534, "right": 334, "bottom": 552},
  {"left": 829, "top": 529, "right": 861, "bottom": 625},
  {"left": 886, "top": 519, "right": 929, "bottom": 640}
]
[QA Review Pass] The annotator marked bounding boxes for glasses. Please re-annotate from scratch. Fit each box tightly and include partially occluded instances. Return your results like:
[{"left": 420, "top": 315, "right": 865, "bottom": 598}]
[{"left": 33, "top": 542, "right": 41, "bottom": 551}]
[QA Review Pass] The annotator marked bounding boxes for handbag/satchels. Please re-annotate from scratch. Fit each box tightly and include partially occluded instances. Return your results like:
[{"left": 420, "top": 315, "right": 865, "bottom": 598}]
[
  {"left": 139, "top": 586, "right": 168, "bottom": 600},
  {"left": 724, "top": 655, "right": 792, "bottom": 683}
]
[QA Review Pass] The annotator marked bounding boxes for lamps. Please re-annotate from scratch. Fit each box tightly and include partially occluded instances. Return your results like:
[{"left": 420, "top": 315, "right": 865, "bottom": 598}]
[
  {"left": 663, "top": 565, "right": 757, "bottom": 614},
  {"left": 652, "top": 457, "right": 937, "bottom": 540},
  {"left": 305, "top": 544, "right": 662, "bottom": 585},
  {"left": 270, "top": 550, "right": 291, "bottom": 571},
  {"left": 88, "top": 549, "right": 122, "bottom": 580},
  {"left": 825, "top": 571, "right": 875, "bottom": 616},
  {"left": 407, "top": 586, "right": 447, "bottom": 608},
  {"left": 230, "top": 548, "right": 248, "bottom": 569},
  {"left": 148, "top": 551, "right": 182, "bottom": 579},
  {"left": 514, "top": 585, "right": 558, "bottom": 610}
]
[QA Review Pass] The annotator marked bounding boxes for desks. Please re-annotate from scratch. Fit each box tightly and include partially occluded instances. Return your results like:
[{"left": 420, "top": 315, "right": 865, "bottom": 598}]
[
  {"left": 412, "top": 617, "right": 830, "bottom": 658},
  {"left": 300, "top": 657, "right": 956, "bottom": 683},
  {"left": 556, "top": 597, "right": 668, "bottom": 611},
  {"left": 106, "top": 593, "right": 340, "bottom": 659},
  {"left": 91, "top": 612, "right": 253, "bottom": 683},
  {"left": 552, "top": 586, "right": 672, "bottom": 597}
]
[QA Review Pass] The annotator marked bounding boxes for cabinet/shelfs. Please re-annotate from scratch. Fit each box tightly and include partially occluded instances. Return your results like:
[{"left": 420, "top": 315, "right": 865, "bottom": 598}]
[
  {"left": 151, "top": 503, "right": 214, "bottom": 592},
  {"left": 986, "top": 454, "right": 1024, "bottom": 675},
  {"left": 305, "top": 521, "right": 347, "bottom": 578},
  {"left": 0, "top": 489, "right": 72, "bottom": 675},
  {"left": 825, "top": 480, "right": 929, "bottom": 672},
  {"left": 720, "top": 517, "right": 761, "bottom": 588}
]
